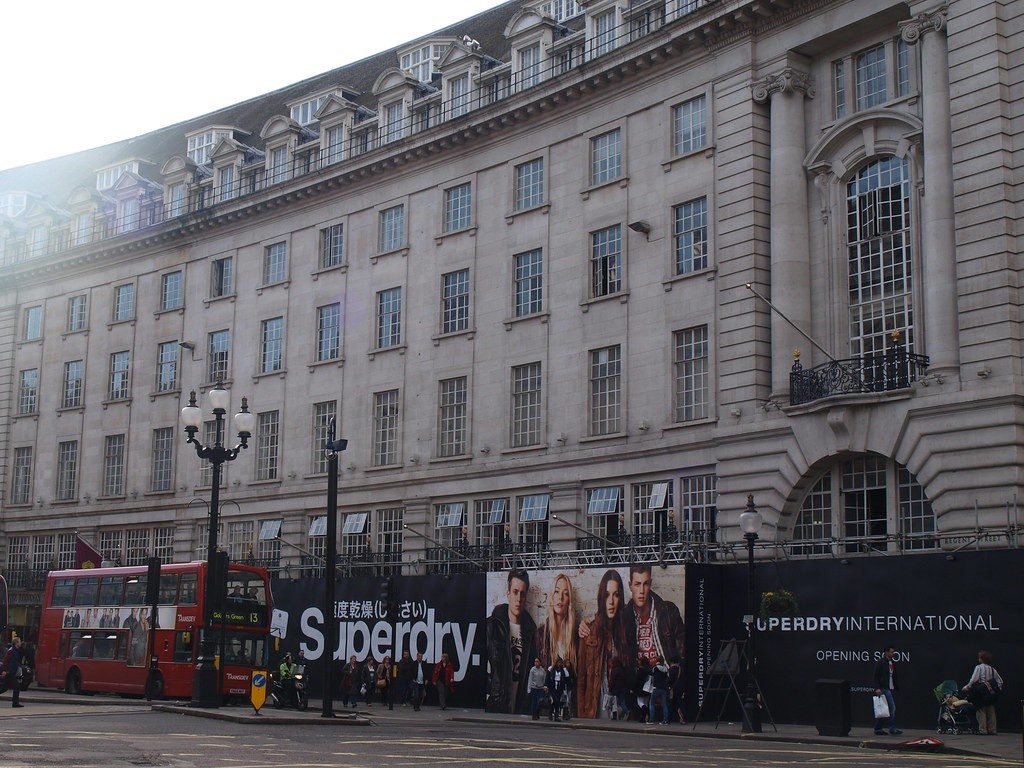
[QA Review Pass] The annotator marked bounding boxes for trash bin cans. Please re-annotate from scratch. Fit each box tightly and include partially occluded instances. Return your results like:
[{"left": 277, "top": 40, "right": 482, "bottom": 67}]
[{"left": 813, "top": 679, "right": 851, "bottom": 737}]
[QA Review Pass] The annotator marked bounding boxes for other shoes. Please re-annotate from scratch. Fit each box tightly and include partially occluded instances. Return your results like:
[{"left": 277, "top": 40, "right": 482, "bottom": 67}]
[
  {"left": 645, "top": 722, "right": 655, "bottom": 725},
  {"left": 414, "top": 707, "right": 421, "bottom": 711},
  {"left": 367, "top": 703, "right": 372, "bottom": 707},
  {"left": 973, "top": 730, "right": 989, "bottom": 736},
  {"left": 549, "top": 715, "right": 553, "bottom": 721},
  {"left": 874, "top": 731, "right": 888, "bottom": 735},
  {"left": 344, "top": 704, "right": 348, "bottom": 708},
  {"left": 890, "top": 729, "right": 903, "bottom": 734},
  {"left": 658, "top": 722, "right": 669, "bottom": 726},
  {"left": 624, "top": 709, "right": 631, "bottom": 721},
  {"left": 680, "top": 721, "right": 687, "bottom": 725},
  {"left": 14, "top": 703, "right": 24, "bottom": 707},
  {"left": 642, "top": 706, "right": 647, "bottom": 717},
  {"left": 442, "top": 705, "right": 446, "bottom": 710},
  {"left": 352, "top": 703, "right": 357, "bottom": 708},
  {"left": 564, "top": 714, "right": 570, "bottom": 721},
  {"left": 554, "top": 718, "right": 562, "bottom": 722},
  {"left": 640, "top": 718, "right": 646, "bottom": 723},
  {"left": 532, "top": 715, "right": 541, "bottom": 720}
]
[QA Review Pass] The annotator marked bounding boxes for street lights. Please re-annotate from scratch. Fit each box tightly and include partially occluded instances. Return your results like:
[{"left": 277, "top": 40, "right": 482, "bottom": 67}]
[
  {"left": 181, "top": 373, "right": 258, "bottom": 705},
  {"left": 737, "top": 490, "right": 762, "bottom": 733},
  {"left": 319, "top": 417, "right": 349, "bottom": 718}
]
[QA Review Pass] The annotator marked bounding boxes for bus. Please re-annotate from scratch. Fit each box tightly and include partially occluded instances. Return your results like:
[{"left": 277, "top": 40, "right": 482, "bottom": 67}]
[{"left": 33, "top": 560, "right": 274, "bottom": 702}]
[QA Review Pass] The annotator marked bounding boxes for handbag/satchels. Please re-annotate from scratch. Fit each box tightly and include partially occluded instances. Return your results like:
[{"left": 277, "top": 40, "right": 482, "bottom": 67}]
[
  {"left": 872, "top": 694, "right": 890, "bottom": 718},
  {"left": 643, "top": 674, "right": 654, "bottom": 694},
  {"left": 376, "top": 676, "right": 387, "bottom": 688},
  {"left": 344, "top": 678, "right": 352, "bottom": 688},
  {"left": 359, "top": 684, "right": 368, "bottom": 695},
  {"left": 449, "top": 685, "right": 457, "bottom": 694},
  {"left": 982, "top": 678, "right": 1001, "bottom": 705},
  {"left": 668, "top": 686, "right": 676, "bottom": 701},
  {"left": 602, "top": 694, "right": 617, "bottom": 712}
]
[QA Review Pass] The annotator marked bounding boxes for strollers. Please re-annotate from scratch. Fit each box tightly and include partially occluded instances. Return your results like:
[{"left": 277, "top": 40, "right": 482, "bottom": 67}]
[{"left": 933, "top": 678, "right": 980, "bottom": 735}]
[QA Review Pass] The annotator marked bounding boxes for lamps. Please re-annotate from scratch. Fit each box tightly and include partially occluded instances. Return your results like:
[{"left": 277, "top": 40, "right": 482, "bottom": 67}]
[
  {"left": 627, "top": 220, "right": 651, "bottom": 234},
  {"left": 178, "top": 341, "right": 195, "bottom": 350}
]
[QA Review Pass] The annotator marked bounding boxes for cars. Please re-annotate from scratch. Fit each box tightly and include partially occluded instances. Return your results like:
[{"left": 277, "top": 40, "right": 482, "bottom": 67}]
[{"left": 0, "top": 642, "right": 33, "bottom": 694}]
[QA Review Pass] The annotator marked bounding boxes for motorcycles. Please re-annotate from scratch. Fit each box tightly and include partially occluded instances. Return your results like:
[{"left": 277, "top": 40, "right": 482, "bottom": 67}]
[{"left": 270, "top": 669, "right": 310, "bottom": 711}]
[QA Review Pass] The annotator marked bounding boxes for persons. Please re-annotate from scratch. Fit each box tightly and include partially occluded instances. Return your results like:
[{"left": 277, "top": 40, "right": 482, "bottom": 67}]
[
  {"left": 873, "top": 645, "right": 903, "bottom": 736},
  {"left": 228, "top": 585, "right": 258, "bottom": 604},
  {"left": 432, "top": 653, "right": 455, "bottom": 710},
  {"left": 361, "top": 656, "right": 378, "bottom": 707},
  {"left": 376, "top": 656, "right": 392, "bottom": 706},
  {"left": 486, "top": 571, "right": 538, "bottom": 714},
  {"left": 607, "top": 656, "right": 687, "bottom": 726},
  {"left": 64, "top": 608, "right": 120, "bottom": 628},
  {"left": 279, "top": 650, "right": 311, "bottom": 698},
  {"left": 342, "top": 655, "right": 360, "bottom": 708},
  {"left": 408, "top": 652, "right": 429, "bottom": 711},
  {"left": 123, "top": 608, "right": 150, "bottom": 666},
  {"left": 579, "top": 563, "right": 684, "bottom": 664},
  {"left": 526, "top": 657, "right": 576, "bottom": 721},
  {"left": 945, "top": 694, "right": 956, "bottom": 708},
  {"left": 961, "top": 650, "right": 1004, "bottom": 735},
  {"left": 576, "top": 570, "right": 635, "bottom": 721},
  {"left": 0, "top": 637, "right": 35, "bottom": 708},
  {"left": 536, "top": 574, "right": 581, "bottom": 666},
  {"left": 398, "top": 650, "right": 414, "bottom": 706}
]
